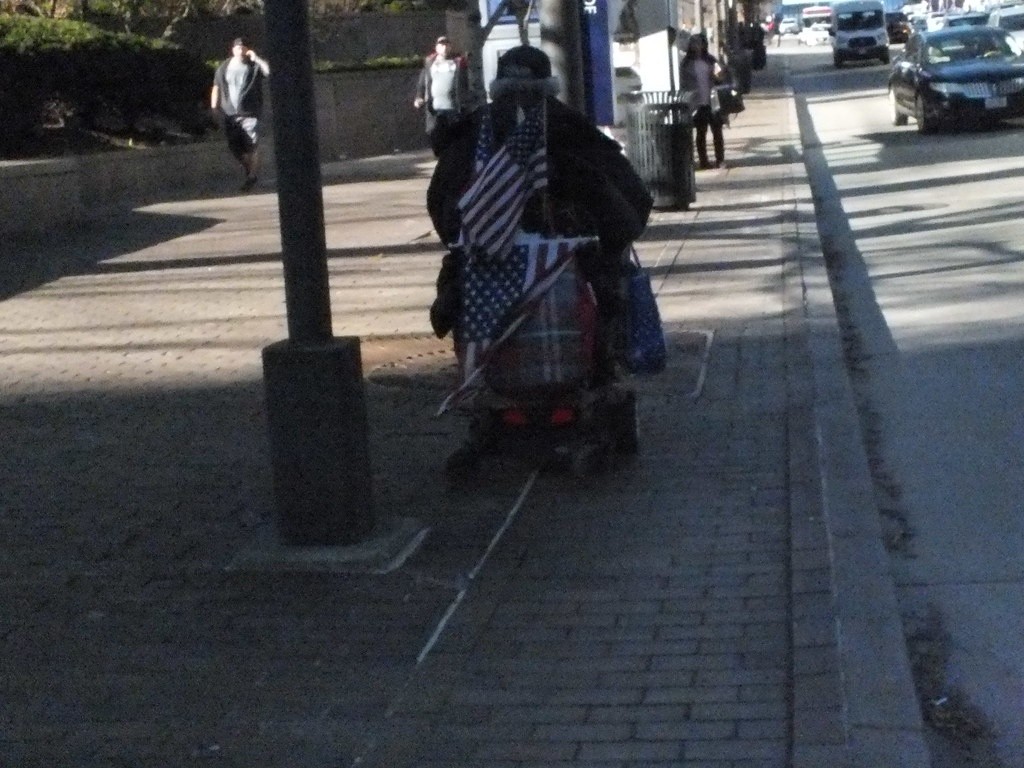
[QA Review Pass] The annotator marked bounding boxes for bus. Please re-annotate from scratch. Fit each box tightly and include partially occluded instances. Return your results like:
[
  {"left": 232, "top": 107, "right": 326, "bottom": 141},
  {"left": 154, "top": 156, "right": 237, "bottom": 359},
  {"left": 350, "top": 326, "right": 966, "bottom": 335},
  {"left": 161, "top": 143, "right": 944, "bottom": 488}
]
[
  {"left": 802, "top": 4, "right": 834, "bottom": 38},
  {"left": 828, "top": 2, "right": 890, "bottom": 69}
]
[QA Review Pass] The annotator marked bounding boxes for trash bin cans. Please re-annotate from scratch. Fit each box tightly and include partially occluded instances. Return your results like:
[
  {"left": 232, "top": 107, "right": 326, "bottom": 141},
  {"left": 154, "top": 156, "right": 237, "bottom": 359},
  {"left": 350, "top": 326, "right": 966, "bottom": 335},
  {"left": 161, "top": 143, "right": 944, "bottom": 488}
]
[
  {"left": 625, "top": 101, "right": 696, "bottom": 212},
  {"left": 728, "top": 53, "right": 752, "bottom": 95}
]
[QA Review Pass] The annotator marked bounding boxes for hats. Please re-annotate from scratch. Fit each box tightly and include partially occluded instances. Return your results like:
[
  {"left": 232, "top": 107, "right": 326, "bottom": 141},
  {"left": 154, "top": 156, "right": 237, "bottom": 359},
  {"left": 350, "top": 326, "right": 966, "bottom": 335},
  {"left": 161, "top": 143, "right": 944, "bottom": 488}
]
[
  {"left": 489, "top": 44, "right": 561, "bottom": 100},
  {"left": 437, "top": 36, "right": 451, "bottom": 44}
]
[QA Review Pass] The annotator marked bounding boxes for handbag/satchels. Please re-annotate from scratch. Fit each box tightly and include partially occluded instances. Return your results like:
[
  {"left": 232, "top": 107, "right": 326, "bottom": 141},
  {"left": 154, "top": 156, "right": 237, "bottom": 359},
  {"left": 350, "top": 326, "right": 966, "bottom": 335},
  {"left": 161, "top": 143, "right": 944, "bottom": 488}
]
[
  {"left": 485, "top": 238, "right": 667, "bottom": 398},
  {"left": 710, "top": 71, "right": 744, "bottom": 115}
]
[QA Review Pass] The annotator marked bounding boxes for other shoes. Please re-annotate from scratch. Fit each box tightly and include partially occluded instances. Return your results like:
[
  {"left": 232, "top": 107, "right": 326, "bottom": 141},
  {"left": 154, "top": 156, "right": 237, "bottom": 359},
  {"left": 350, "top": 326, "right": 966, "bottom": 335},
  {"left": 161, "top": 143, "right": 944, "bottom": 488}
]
[
  {"left": 241, "top": 175, "right": 260, "bottom": 190},
  {"left": 700, "top": 160, "right": 714, "bottom": 169},
  {"left": 717, "top": 162, "right": 728, "bottom": 169}
]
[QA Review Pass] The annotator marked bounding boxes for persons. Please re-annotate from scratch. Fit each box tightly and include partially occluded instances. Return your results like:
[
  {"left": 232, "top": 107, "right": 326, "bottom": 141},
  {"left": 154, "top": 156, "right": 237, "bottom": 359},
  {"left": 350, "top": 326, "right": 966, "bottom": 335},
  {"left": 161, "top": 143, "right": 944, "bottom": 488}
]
[
  {"left": 210, "top": 37, "right": 270, "bottom": 193},
  {"left": 413, "top": 36, "right": 470, "bottom": 135},
  {"left": 428, "top": 46, "right": 655, "bottom": 340},
  {"left": 678, "top": 33, "right": 729, "bottom": 169},
  {"left": 739, "top": 19, "right": 764, "bottom": 47}
]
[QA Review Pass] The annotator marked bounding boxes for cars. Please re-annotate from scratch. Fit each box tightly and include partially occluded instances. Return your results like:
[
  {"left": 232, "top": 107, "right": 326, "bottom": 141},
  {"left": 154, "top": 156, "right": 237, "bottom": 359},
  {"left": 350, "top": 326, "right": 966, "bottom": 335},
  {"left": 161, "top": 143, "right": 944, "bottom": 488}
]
[
  {"left": 885, "top": 12, "right": 915, "bottom": 44},
  {"left": 901, "top": 3, "right": 943, "bottom": 33},
  {"left": 779, "top": 16, "right": 799, "bottom": 35},
  {"left": 943, "top": 12, "right": 989, "bottom": 28},
  {"left": 888, "top": 27, "right": 1024, "bottom": 135},
  {"left": 988, "top": 5, "right": 1024, "bottom": 53}
]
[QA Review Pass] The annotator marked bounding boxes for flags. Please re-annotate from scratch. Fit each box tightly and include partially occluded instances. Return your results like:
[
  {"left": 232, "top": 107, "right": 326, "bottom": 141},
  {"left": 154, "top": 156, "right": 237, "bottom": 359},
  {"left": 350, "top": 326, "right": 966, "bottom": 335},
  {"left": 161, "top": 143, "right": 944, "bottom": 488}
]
[
  {"left": 457, "top": 240, "right": 579, "bottom": 391},
  {"left": 457, "top": 103, "right": 547, "bottom": 262}
]
